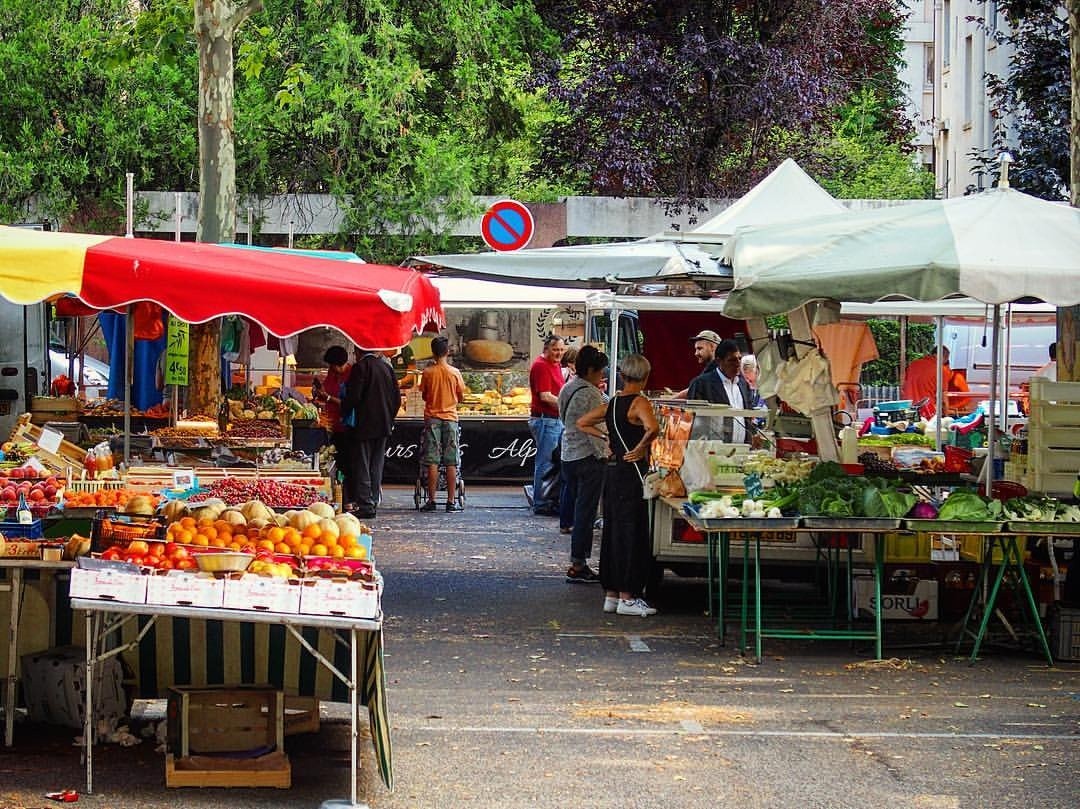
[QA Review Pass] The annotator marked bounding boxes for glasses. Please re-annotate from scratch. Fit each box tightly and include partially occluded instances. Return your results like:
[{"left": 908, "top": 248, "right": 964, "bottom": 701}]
[{"left": 590, "top": 350, "right": 601, "bottom": 368}]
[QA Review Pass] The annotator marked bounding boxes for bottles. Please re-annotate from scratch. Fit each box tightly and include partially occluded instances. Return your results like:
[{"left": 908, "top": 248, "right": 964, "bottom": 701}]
[
  {"left": 218, "top": 394, "right": 229, "bottom": 432},
  {"left": 16, "top": 492, "right": 32, "bottom": 525},
  {"left": 84, "top": 446, "right": 113, "bottom": 480}
]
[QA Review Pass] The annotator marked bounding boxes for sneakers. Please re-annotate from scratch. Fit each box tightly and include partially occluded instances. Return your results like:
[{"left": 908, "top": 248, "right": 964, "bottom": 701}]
[
  {"left": 603, "top": 596, "right": 620, "bottom": 612},
  {"left": 616, "top": 598, "right": 657, "bottom": 615},
  {"left": 566, "top": 565, "right": 600, "bottom": 584}
]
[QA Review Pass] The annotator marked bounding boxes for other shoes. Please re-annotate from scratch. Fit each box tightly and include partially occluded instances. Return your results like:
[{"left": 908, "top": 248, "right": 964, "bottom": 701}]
[
  {"left": 560, "top": 527, "right": 573, "bottom": 534},
  {"left": 420, "top": 501, "right": 437, "bottom": 512},
  {"left": 349, "top": 508, "right": 376, "bottom": 519},
  {"left": 343, "top": 503, "right": 355, "bottom": 513},
  {"left": 523, "top": 485, "right": 535, "bottom": 507},
  {"left": 533, "top": 504, "right": 560, "bottom": 516},
  {"left": 446, "top": 502, "right": 464, "bottom": 512}
]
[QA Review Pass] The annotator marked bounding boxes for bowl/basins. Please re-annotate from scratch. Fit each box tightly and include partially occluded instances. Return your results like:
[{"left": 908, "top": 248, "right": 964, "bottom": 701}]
[{"left": 190, "top": 552, "right": 255, "bottom": 571}]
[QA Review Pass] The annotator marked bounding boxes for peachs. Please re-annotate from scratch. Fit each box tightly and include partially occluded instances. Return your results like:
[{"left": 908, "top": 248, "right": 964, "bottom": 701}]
[{"left": 0, "top": 467, "right": 59, "bottom": 503}]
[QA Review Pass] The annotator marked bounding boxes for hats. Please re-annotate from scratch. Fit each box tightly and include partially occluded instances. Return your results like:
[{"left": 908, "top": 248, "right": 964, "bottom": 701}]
[{"left": 688, "top": 330, "right": 722, "bottom": 345}]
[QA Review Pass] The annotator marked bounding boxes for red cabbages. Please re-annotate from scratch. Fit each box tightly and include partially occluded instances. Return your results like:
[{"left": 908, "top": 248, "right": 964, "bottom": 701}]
[{"left": 908, "top": 502, "right": 938, "bottom": 519}]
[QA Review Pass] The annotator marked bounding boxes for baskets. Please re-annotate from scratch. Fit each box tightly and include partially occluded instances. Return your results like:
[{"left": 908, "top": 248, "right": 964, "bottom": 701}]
[
  {"left": 873, "top": 406, "right": 921, "bottom": 428},
  {"left": 0, "top": 503, "right": 57, "bottom": 518},
  {"left": 91, "top": 509, "right": 169, "bottom": 553}
]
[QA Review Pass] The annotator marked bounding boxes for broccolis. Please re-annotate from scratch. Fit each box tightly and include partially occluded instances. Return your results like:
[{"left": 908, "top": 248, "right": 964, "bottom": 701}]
[
  {"left": 796, "top": 459, "right": 856, "bottom": 516},
  {"left": 243, "top": 396, "right": 313, "bottom": 421}
]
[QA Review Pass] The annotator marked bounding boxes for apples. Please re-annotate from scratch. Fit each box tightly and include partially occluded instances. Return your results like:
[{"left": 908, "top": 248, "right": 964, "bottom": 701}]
[{"left": 103, "top": 542, "right": 297, "bottom": 578}]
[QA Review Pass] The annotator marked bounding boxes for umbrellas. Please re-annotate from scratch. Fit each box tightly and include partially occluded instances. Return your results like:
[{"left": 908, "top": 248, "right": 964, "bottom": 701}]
[
  {"left": 0, "top": 174, "right": 445, "bottom": 467},
  {"left": 393, "top": 157, "right": 879, "bottom": 497},
  {"left": 715, "top": 152, "right": 1080, "bottom": 496}
]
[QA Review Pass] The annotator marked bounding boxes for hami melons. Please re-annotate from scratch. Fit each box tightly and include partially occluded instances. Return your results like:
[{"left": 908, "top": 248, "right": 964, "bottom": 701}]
[{"left": 126, "top": 495, "right": 361, "bottom": 537}]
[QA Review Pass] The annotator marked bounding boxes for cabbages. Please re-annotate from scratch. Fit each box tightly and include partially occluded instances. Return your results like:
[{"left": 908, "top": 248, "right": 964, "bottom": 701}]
[{"left": 855, "top": 478, "right": 996, "bottom": 520}]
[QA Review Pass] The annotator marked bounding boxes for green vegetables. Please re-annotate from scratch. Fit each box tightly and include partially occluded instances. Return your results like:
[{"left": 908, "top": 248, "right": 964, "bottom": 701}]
[
  {"left": 857, "top": 433, "right": 937, "bottom": 450},
  {"left": 688, "top": 477, "right": 801, "bottom": 507},
  {"left": 989, "top": 494, "right": 1080, "bottom": 524}
]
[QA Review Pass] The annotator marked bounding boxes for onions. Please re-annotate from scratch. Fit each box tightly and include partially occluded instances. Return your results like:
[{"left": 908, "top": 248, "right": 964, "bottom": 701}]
[{"left": 699, "top": 496, "right": 781, "bottom": 518}]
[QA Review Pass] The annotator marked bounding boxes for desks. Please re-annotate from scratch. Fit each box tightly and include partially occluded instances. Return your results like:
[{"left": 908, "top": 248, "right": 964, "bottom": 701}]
[
  {"left": 72, "top": 598, "right": 382, "bottom": 807},
  {"left": 0, "top": 559, "right": 106, "bottom": 748},
  {"left": 929, "top": 530, "right": 1080, "bottom": 666},
  {"left": 699, "top": 527, "right": 895, "bottom": 663}
]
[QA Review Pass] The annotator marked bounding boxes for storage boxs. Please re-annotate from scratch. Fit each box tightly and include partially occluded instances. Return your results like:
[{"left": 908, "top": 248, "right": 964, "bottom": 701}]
[
  {"left": 962, "top": 534, "right": 1025, "bottom": 565},
  {"left": 872, "top": 374, "right": 1080, "bottom": 497},
  {"left": 1044, "top": 599, "right": 1080, "bottom": 662},
  {"left": 20, "top": 643, "right": 123, "bottom": 728},
  {"left": 885, "top": 531, "right": 931, "bottom": 564},
  {"left": 0, "top": 397, "right": 379, "bottom": 617},
  {"left": 854, "top": 579, "right": 938, "bottom": 620},
  {"left": 164, "top": 679, "right": 320, "bottom": 786}
]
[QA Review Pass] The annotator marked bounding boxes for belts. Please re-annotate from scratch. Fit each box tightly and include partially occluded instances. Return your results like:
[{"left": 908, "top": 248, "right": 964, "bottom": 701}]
[{"left": 531, "top": 413, "right": 559, "bottom": 419}]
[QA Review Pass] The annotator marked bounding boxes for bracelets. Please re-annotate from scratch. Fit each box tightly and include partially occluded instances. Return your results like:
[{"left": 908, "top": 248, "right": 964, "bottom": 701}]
[
  {"left": 326, "top": 395, "right": 331, "bottom": 403},
  {"left": 600, "top": 432, "right": 608, "bottom": 441}
]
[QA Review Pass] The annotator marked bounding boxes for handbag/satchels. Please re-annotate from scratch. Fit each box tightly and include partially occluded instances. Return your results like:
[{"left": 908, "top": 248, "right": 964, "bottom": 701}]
[
  {"left": 339, "top": 382, "right": 356, "bottom": 426},
  {"left": 642, "top": 470, "right": 660, "bottom": 499}
]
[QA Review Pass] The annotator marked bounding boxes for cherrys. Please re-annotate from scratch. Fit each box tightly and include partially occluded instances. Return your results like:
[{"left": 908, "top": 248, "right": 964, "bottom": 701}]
[{"left": 185, "top": 477, "right": 331, "bottom": 506}]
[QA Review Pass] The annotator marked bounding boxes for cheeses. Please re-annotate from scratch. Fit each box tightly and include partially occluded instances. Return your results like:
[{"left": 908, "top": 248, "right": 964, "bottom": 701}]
[{"left": 462, "top": 387, "right": 529, "bottom": 414}]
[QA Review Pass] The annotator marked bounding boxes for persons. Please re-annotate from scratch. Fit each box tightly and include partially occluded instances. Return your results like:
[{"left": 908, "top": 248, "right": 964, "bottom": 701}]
[
  {"left": 671, "top": 329, "right": 769, "bottom": 446},
  {"left": 1032, "top": 342, "right": 1056, "bottom": 381},
  {"left": 310, "top": 336, "right": 401, "bottom": 521},
  {"left": 527, "top": 334, "right": 610, "bottom": 585},
  {"left": 902, "top": 344, "right": 969, "bottom": 421},
  {"left": 576, "top": 354, "right": 660, "bottom": 617},
  {"left": 419, "top": 336, "right": 466, "bottom": 514}
]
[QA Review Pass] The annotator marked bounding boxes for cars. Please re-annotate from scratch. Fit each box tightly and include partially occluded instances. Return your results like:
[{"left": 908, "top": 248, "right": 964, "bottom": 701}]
[{"left": 49, "top": 329, "right": 111, "bottom": 399}]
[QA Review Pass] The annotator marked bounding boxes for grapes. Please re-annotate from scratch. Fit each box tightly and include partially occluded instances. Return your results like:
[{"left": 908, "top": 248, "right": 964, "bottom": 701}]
[{"left": 858, "top": 452, "right": 895, "bottom": 474}]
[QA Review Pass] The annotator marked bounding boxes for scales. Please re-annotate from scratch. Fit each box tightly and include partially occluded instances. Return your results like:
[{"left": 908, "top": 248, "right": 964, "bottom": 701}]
[
  {"left": 40, "top": 507, "right": 117, "bottom": 540},
  {"left": 774, "top": 411, "right": 844, "bottom": 438}
]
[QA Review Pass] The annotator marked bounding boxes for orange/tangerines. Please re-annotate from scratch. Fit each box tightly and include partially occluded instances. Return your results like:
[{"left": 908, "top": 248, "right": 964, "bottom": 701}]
[
  {"left": 166, "top": 516, "right": 366, "bottom": 558},
  {"left": 64, "top": 489, "right": 159, "bottom": 507}
]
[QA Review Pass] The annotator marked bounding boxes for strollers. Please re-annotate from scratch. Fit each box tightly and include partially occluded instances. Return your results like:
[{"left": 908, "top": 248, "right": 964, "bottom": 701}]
[{"left": 413, "top": 420, "right": 467, "bottom": 510}]
